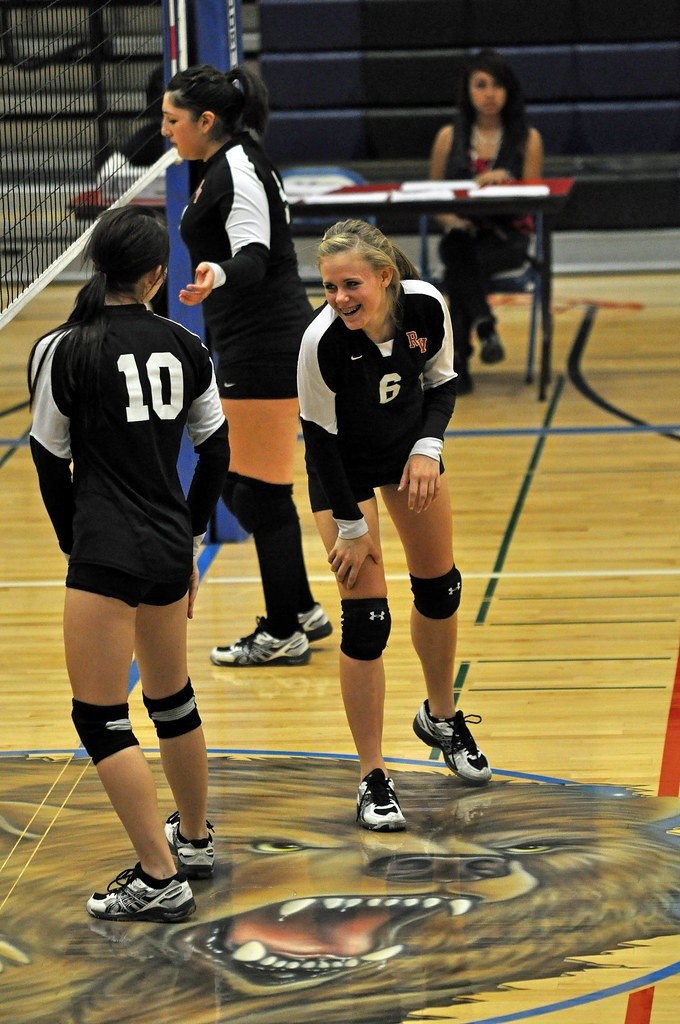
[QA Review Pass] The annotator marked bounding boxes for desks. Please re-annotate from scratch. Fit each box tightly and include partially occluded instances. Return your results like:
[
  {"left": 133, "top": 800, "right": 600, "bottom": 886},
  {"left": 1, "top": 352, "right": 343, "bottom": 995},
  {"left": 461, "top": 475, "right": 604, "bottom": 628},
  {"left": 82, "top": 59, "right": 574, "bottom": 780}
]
[{"left": 69, "top": 176, "right": 575, "bottom": 401}]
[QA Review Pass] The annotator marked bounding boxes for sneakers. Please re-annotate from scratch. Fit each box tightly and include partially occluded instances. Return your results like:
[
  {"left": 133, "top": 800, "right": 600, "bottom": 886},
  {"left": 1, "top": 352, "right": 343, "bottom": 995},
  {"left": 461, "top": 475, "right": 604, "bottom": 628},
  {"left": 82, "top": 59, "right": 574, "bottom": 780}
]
[
  {"left": 165, "top": 810, "right": 216, "bottom": 879},
  {"left": 413, "top": 699, "right": 492, "bottom": 783},
  {"left": 356, "top": 768, "right": 406, "bottom": 831},
  {"left": 86, "top": 862, "right": 196, "bottom": 922},
  {"left": 297, "top": 603, "right": 333, "bottom": 642},
  {"left": 210, "top": 616, "right": 310, "bottom": 667}
]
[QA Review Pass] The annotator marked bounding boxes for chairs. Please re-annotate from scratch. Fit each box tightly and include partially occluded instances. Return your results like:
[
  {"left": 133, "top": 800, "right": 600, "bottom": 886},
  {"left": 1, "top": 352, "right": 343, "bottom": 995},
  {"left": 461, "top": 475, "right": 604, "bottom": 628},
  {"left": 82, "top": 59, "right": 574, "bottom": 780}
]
[
  {"left": 421, "top": 208, "right": 543, "bottom": 383},
  {"left": 282, "top": 167, "right": 377, "bottom": 290}
]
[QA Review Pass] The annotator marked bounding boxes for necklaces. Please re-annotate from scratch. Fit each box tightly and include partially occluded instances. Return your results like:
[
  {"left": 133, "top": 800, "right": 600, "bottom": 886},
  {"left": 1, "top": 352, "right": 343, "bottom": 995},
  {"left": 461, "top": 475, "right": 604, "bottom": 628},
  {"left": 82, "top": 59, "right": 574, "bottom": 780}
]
[{"left": 471, "top": 125, "right": 502, "bottom": 146}]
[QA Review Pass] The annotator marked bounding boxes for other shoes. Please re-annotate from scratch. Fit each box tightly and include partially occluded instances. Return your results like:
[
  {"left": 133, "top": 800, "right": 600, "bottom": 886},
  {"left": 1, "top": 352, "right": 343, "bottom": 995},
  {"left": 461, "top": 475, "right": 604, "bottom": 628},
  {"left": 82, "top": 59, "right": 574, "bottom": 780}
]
[
  {"left": 453, "top": 364, "right": 473, "bottom": 394},
  {"left": 480, "top": 332, "right": 503, "bottom": 363}
]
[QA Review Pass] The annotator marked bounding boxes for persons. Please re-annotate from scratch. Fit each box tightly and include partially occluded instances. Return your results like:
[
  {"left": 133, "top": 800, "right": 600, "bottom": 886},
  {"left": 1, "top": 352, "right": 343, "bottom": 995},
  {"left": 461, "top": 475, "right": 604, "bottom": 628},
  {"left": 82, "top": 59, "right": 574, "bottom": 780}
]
[
  {"left": 25, "top": 202, "right": 214, "bottom": 925},
  {"left": 161, "top": 64, "right": 333, "bottom": 666},
  {"left": 297, "top": 221, "right": 493, "bottom": 831},
  {"left": 429, "top": 49, "right": 542, "bottom": 395}
]
